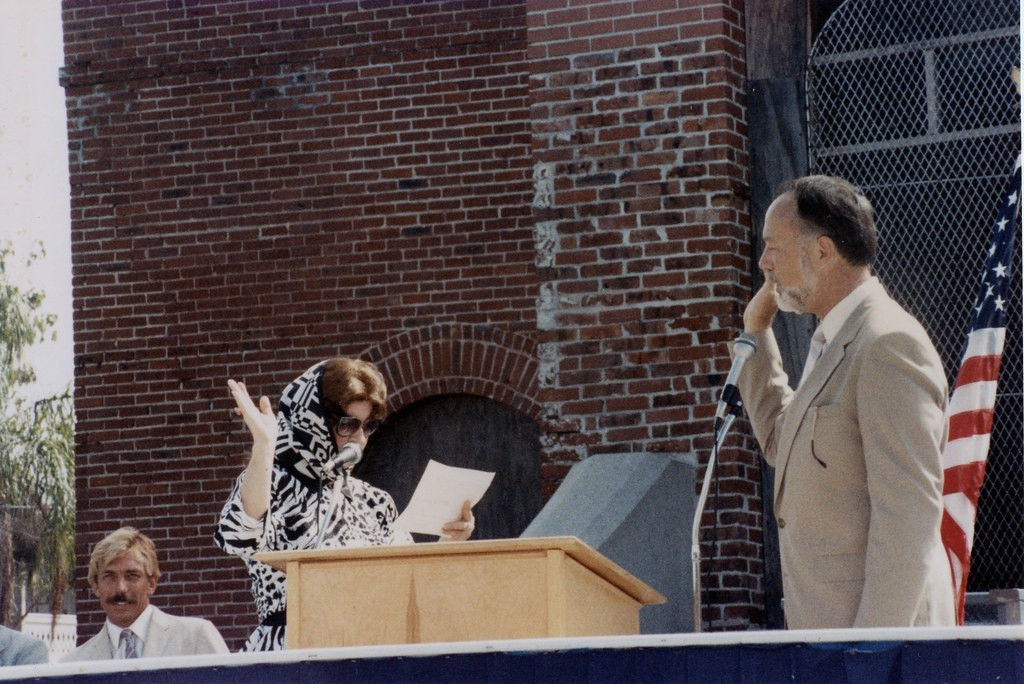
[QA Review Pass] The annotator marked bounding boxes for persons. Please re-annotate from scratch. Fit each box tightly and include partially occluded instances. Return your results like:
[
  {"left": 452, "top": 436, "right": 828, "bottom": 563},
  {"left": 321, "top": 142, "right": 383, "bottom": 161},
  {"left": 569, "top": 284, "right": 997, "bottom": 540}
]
[
  {"left": 727, "top": 174, "right": 956, "bottom": 629},
  {"left": 212, "top": 354, "right": 478, "bottom": 653},
  {"left": 46, "top": 524, "right": 231, "bottom": 664},
  {"left": 0, "top": 622, "right": 46, "bottom": 665}
]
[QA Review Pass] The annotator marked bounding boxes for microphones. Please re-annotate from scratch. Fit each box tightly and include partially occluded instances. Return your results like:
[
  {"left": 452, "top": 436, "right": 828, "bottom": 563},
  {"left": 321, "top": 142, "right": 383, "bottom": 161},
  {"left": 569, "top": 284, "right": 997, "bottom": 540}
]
[
  {"left": 713, "top": 332, "right": 759, "bottom": 430},
  {"left": 320, "top": 441, "right": 364, "bottom": 476}
]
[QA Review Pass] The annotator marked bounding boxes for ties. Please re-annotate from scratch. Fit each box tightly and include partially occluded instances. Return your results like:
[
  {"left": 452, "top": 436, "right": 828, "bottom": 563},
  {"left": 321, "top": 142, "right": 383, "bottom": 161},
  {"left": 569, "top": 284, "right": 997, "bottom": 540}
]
[
  {"left": 797, "top": 325, "right": 824, "bottom": 394},
  {"left": 120, "top": 628, "right": 138, "bottom": 659}
]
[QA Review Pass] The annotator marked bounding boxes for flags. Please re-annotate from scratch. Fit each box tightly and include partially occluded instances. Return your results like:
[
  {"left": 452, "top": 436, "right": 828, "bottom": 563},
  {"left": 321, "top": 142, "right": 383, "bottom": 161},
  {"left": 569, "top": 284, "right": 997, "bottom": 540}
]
[{"left": 936, "top": 149, "right": 1022, "bottom": 627}]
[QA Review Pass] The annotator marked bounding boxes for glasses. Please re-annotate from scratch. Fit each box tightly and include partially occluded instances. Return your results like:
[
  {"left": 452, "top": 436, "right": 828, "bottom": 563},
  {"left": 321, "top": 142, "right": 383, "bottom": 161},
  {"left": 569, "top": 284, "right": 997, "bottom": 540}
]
[{"left": 331, "top": 412, "right": 381, "bottom": 438}]
